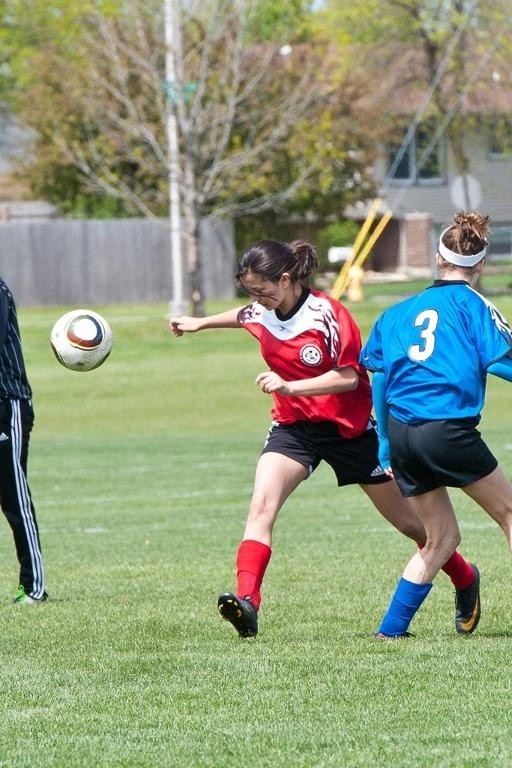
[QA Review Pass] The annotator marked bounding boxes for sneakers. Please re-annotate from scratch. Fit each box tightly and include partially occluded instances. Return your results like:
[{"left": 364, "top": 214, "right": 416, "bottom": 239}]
[
  {"left": 375, "top": 631, "right": 417, "bottom": 641},
  {"left": 451, "top": 562, "right": 481, "bottom": 635},
  {"left": 11, "top": 590, "right": 50, "bottom": 605},
  {"left": 218, "top": 590, "right": 258, "bottom": 638}
]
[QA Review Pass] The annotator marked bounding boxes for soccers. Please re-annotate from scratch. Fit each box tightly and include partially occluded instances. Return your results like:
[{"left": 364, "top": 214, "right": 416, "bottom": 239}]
[{"left": 48, "top": 308, "right": 114, "bottom": 372}]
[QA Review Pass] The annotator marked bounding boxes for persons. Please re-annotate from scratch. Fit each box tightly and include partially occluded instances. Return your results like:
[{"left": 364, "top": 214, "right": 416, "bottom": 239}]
[
  {"left": 0, "top": 275, "right": 49, "bottom": 604},
  {"left": 358, "top": 206, "right": 512, "bottom": 640},
  {"left": 166, "top": 236, "right": 483, "bottom": 639}
]
